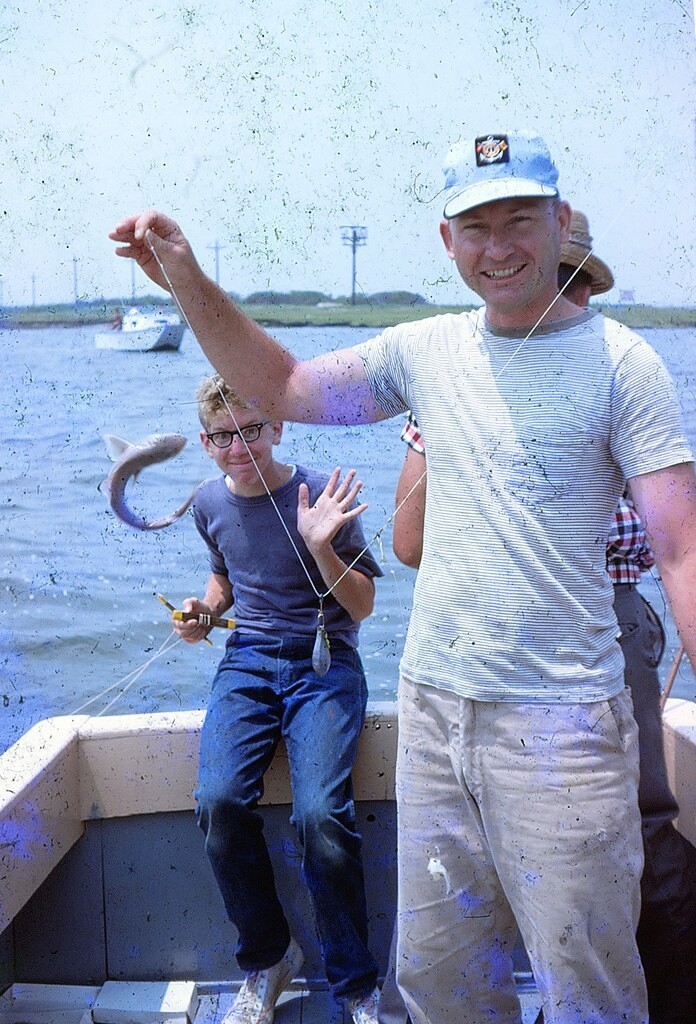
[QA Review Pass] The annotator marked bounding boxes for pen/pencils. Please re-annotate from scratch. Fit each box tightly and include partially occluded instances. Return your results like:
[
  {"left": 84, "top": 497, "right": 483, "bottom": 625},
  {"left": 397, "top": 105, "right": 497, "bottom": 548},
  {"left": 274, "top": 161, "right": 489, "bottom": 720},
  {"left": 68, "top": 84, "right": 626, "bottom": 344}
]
[{"left": 156, "top": 593, "right": 214, "bottom": 647}]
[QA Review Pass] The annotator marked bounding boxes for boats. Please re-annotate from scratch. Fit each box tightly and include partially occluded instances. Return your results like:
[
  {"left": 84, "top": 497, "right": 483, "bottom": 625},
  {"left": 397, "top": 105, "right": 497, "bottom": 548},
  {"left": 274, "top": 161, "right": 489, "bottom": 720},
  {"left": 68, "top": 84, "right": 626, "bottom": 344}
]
[{"left": 95, "top": 307, "right": 187, "bottom": 354}]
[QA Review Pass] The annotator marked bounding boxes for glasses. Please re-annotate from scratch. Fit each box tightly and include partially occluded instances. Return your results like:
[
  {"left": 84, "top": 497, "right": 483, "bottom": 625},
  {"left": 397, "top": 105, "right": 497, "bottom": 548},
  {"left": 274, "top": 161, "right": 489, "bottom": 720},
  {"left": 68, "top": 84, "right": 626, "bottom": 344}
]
[{"left": 205, "top": 420, "right": 270, "bottom": 449}]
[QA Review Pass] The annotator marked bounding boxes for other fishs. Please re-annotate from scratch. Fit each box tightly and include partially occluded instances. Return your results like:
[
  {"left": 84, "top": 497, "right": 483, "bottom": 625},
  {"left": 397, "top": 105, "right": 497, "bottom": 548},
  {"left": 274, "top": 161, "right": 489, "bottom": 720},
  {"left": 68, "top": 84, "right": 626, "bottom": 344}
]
[{"left": 98, "top": 433, "right": 195, "bottom": 530}]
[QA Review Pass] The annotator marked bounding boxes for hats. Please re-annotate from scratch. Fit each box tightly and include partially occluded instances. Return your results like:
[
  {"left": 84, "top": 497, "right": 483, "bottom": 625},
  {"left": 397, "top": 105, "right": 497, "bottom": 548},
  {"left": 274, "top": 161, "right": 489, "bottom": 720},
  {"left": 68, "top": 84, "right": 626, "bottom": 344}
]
[
  {"left": 560, "top": 210, "right": 614, "bottom": 296},
  {"left": 442, "top": 129, "right": 559, "bottom": 219}
]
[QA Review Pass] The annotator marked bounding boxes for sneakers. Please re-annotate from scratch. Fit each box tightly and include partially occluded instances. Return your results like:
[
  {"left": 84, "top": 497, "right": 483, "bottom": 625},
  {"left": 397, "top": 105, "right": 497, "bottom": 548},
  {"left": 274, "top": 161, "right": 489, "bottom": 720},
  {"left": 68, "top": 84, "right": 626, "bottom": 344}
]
[
  {"left": 221, "top": 937, "right": 305, "bottom": 1024},
  {"left": 344, "top": 985, "right": 380, "bottom": 1024}
]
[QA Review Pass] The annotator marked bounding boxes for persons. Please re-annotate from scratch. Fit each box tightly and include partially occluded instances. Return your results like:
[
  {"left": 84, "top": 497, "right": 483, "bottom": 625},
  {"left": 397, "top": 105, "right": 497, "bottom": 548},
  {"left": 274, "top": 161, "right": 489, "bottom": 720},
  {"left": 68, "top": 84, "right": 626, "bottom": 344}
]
[
  {"left": 110, "top": 125, "right": 695, "bottom": 1024},
  {"left": 170, "top": 376, "right": 385, "bottom": 1024},
  {"left": 391, "top": 210, "right": 696, "bottom": 1024}
]
